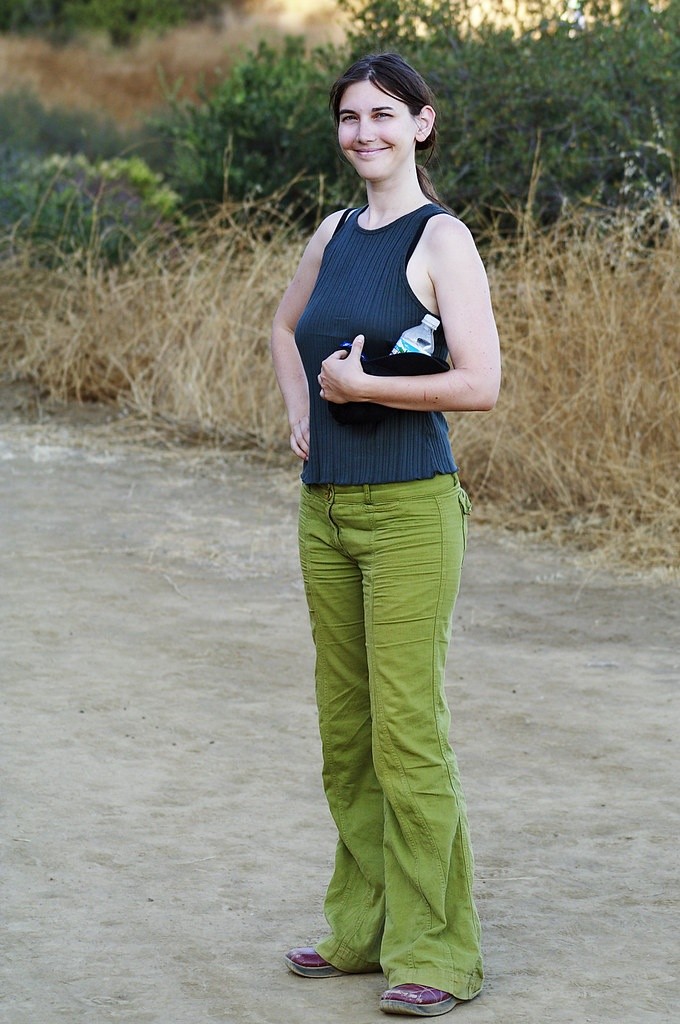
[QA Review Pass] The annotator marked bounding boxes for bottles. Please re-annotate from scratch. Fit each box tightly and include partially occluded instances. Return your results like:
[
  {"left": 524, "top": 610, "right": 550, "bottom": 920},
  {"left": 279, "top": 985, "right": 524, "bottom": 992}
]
[{"left": 390, "top": 313, "right": 441, "bottom": 362}]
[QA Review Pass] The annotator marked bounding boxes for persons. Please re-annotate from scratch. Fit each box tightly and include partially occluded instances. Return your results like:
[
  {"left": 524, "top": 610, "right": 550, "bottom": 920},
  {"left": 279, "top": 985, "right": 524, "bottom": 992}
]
[{"left": 272, "top": 50, "right": 504, "bottom": 1019}]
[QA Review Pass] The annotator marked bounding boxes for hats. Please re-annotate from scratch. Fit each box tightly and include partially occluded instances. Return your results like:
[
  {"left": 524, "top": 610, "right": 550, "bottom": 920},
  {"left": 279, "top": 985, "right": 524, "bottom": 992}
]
[{"left": 327, "top": 338, "right": 451, "bottom": 426}]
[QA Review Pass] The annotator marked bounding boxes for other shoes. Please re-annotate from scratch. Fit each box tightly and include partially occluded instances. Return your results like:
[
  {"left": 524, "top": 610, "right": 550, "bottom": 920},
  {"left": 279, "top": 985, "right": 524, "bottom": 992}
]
[
  {"left": 284, "top": 947, "right": 352, "bottom": 977},
  {"left": 377, "top": 982, "right": 468, "bottom": 1016}
]
